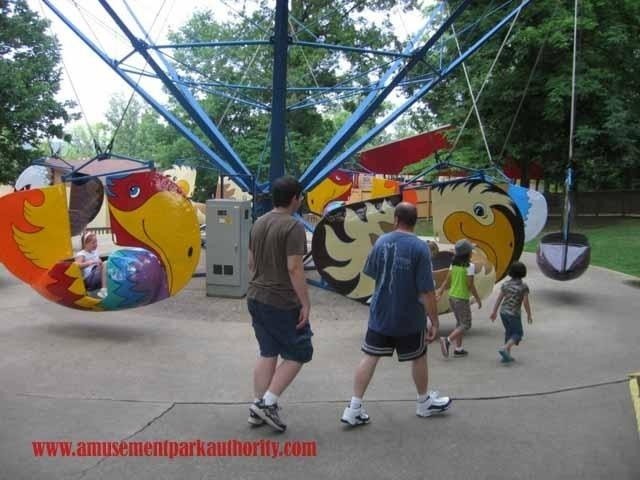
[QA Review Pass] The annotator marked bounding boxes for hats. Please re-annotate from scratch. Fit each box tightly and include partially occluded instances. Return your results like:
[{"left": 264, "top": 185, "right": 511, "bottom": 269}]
[{"left": 455, "top": 237, "right": 477, "bottom": 256}]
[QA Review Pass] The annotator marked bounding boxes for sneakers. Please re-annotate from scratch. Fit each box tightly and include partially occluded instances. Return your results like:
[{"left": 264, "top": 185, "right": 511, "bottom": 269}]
[
  {"left": 454, "top": 348, "right": 469, "bottom": 358},
  {"left": 415, "top": 392, "right": 453, "bottom": 418},
  {"left": 248, "top": 395, "right": 288, "bottom": 434},
  {"left": 498, "top": 348, "right": 514, "bottom": 363},
  {"left": 96, "top": 287, "right": 108, "bottom": 299},
  {"left": 340, "top": 403, "right": 370, "bottom": 426},
  {"left": 439, "top": 335, "right": 450, "bottom": 357}
]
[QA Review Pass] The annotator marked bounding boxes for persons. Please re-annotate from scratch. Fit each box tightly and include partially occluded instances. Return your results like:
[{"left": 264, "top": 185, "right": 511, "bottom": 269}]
[
  {"left": 433, "top": 238, "right": 484, "bottom": 359},
  {"left": 0, "top": 149, "right": 552, "bottom": 314},
  {"left": 488, "top": 260, "right": 535, "bottom": 364},
  {"left": 339, "top": 199, "right": 453, "bottom": 429},
  {"left": 244, "top": 174, "right": 317, "bottom": 434}
]
